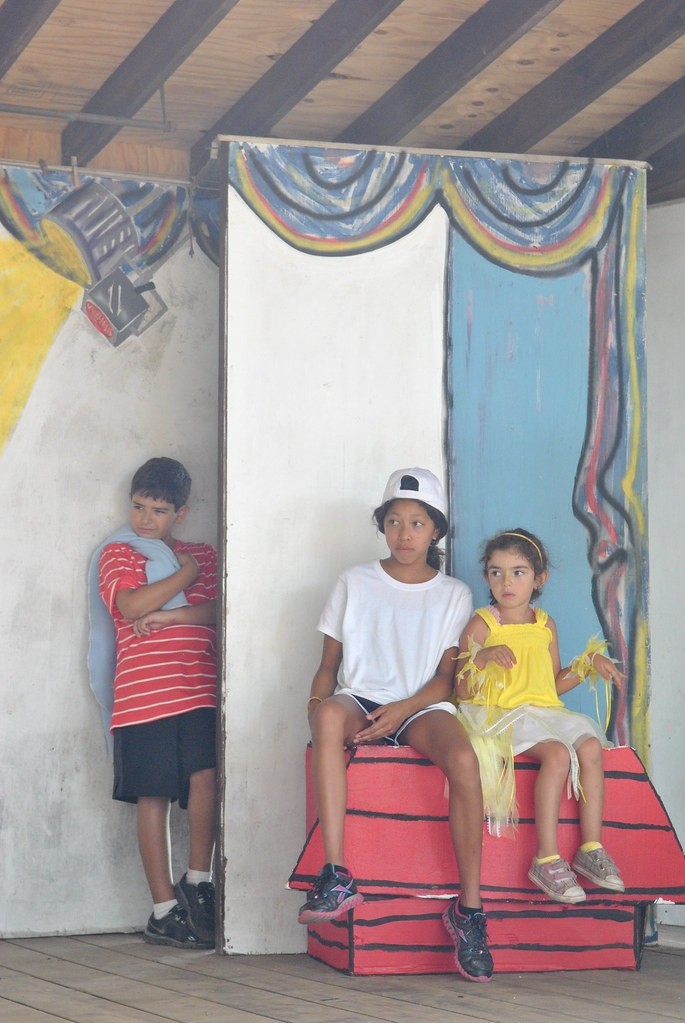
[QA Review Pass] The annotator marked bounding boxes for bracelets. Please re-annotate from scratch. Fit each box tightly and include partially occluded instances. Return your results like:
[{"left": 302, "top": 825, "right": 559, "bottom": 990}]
[{"left": 307, "top": 696, "right": 322, "bottom": 706}]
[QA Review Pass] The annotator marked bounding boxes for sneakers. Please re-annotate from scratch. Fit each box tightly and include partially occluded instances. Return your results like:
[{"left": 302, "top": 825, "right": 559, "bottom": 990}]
[
  {"left": 143, "top": 903, "right": 215, "bottom": 949},
  {"left": 573, "top": 848, "right": 626, "bottom": 893},
  {"left": 174, "top": 872, "right": 215, "bottom": 942},
  {"left": 298, "top": 862, "right": 364, "bottom": 924},
  {"left": 528, "top": 858, "right": 586, "bottom": 903},
  {"left": 442, "top": 892, "right": 494, "bottom": 983}
]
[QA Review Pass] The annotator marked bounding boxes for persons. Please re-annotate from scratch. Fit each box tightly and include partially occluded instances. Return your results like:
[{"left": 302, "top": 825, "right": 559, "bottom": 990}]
[
  {"left": 298, "top": 466, "right": 629, "bottom": 985},
  {"left": 100, "top": 456, "right": 219, "bottom": 948}
]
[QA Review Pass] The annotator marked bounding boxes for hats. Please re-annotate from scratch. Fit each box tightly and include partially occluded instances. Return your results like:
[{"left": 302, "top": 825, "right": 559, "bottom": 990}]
[{"left": 382, "top": 468, "right": 448, "bottom": 518}]
